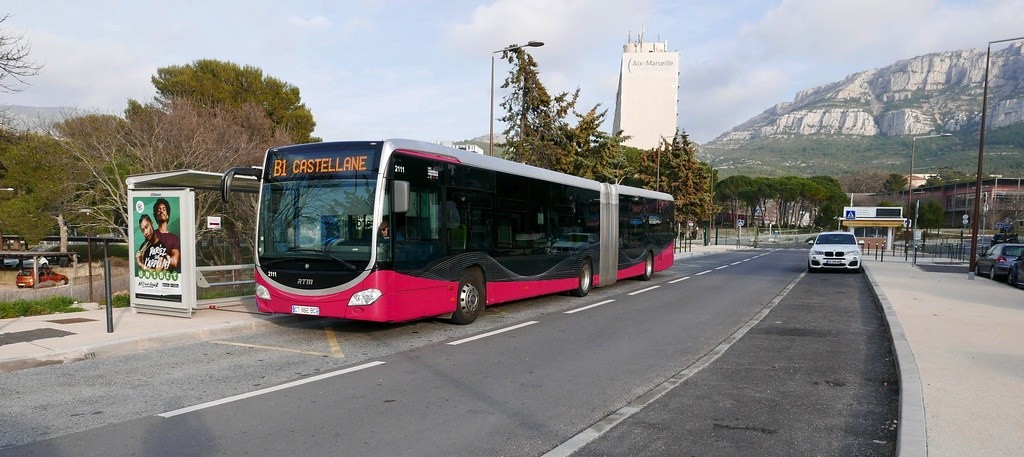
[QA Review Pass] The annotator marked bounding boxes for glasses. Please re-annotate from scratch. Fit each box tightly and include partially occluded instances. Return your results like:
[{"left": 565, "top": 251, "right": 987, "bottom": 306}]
[{"left": 379, "top": 225, "right": 388, "bottom": 231}]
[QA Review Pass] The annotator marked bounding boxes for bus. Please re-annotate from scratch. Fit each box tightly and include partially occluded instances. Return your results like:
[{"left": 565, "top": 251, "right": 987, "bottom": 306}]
[{"left": 219, "top": 136, "right": 677, "bottom": 325}]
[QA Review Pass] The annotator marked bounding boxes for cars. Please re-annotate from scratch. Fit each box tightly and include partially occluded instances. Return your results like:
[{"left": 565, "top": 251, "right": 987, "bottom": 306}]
[
  {"left": 3, "top": 256, "right": 49, "bottom": 270},
  {"left": 805, "top": 232, "right": 862, "bottom": 271},
  {"left": 1008, "top": 251, "right": 1024, "bottom": 289},
  {"left": 975, "top": 243, "right": 1024, "bottom": 281},
  {"left": 990, "top": 233, "right": 1019, "bottom": 248},
  {"left": 15, "top": 267, "right": 68, "bottom": 289}
]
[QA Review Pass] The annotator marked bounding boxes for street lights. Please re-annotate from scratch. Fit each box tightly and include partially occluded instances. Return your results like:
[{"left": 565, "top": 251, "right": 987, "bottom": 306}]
[
  {"left": 489, "top": 41, "right": 546, "bottom": 157},
  {"left": 657, "top": 133, "right": 690, "bottom": 192},
  {"left": 905, "top": 133, "right": 953, "bottom": 262}
]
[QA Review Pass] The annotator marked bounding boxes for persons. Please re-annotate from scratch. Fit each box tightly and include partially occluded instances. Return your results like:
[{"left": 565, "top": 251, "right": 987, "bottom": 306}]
[
  {"left": 136, "top": 214, "right": 166, "bottom": 274},
  {"left": 378, "top": 221, "right": 390, "bottom": 242},
  {"left": 145, "top": 198, "right": 181, "bottom": 274}
]
